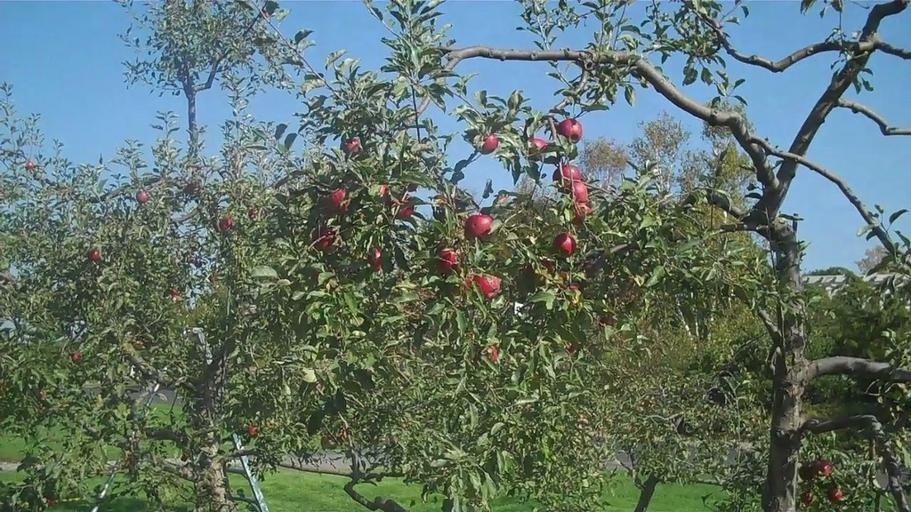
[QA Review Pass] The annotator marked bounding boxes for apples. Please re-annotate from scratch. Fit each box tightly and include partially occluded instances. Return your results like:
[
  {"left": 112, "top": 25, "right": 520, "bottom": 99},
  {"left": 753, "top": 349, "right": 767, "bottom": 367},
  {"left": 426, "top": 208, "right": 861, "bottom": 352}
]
[
  {"left": 247, "top": 425, "right": 259, "bottom": 437},
  {"left": 22, "top": 117, "right": 616, "bottom": 368},
  {"left": 795, "top": 460, "right": 843, "bottom": 504}
]
